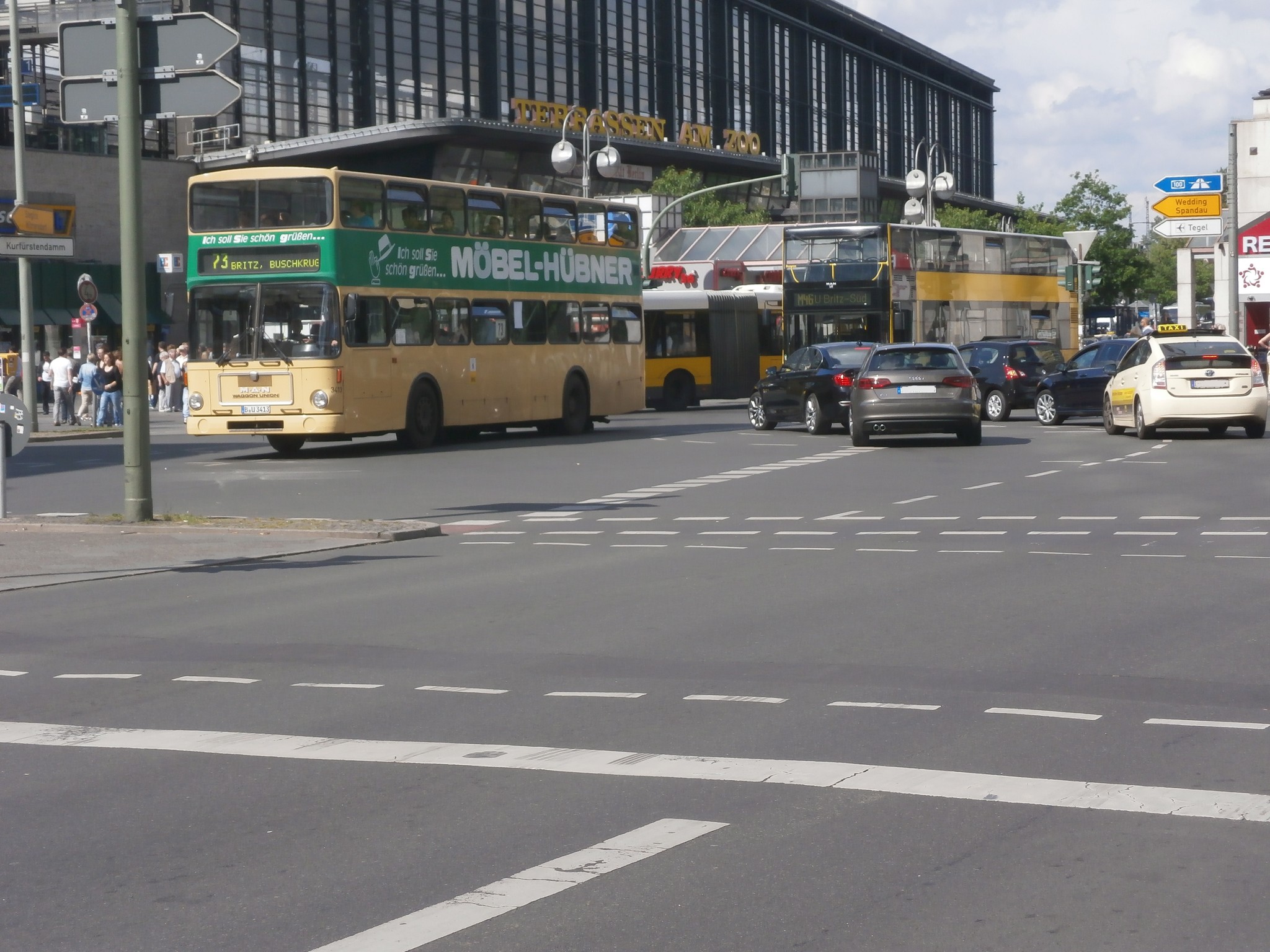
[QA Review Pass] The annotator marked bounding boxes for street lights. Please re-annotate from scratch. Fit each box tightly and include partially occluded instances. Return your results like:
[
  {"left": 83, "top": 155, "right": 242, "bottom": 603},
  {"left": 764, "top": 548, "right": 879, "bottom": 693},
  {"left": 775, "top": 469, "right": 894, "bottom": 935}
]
[{"left": 550, "top": 108, "right": 624, "bottom": 198}]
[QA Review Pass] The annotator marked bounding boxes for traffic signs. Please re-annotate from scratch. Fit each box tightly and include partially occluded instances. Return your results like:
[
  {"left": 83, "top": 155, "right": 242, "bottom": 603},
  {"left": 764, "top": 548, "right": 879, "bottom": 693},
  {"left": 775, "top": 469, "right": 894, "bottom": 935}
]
[
  {"left": 1150, "top": 193, "right": 1222, "bottom": 218},
  {"left": 6, "top": 205, "right": 65, "bottom": 236},
  {"left": 1152, "top": 172, "right": 1224, "bottom": 194},
  {"left": 1152, "top": 216, "right": 1224, "bottom": 238}
]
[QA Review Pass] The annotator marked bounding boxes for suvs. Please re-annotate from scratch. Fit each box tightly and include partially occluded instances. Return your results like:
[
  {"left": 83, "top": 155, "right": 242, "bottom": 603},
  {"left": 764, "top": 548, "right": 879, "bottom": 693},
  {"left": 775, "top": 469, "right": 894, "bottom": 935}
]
[{"left": 841, "top": 339, "right": 983, "bottom": 449}]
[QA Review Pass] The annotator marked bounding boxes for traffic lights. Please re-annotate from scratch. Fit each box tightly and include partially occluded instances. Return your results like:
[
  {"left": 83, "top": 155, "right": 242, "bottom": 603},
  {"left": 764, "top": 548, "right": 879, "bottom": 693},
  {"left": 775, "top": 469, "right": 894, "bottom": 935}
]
[
  {"left": 1057, "top": 263, "right": 1077, "bottom": 292},
  {"left": 1084, "top": 264, "right": 1104, "bottom": 293}
]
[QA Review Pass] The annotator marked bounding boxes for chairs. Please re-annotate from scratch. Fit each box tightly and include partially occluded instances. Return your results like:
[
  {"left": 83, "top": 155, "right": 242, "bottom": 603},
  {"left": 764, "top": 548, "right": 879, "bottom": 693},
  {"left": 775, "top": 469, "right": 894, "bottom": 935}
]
[
  {"left": 979, "top": 348, "right": 1038, "bottom": 367},
  {"left": 880, "top": 353, "right": 949, "bottom": 368}
]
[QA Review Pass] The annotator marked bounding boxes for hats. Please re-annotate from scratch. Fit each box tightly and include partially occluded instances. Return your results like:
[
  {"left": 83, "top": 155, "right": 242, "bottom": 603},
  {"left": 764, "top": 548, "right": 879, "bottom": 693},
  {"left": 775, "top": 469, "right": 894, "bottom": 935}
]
[{"left": 178, "top": 345, "right": 185, "bottom": 350}]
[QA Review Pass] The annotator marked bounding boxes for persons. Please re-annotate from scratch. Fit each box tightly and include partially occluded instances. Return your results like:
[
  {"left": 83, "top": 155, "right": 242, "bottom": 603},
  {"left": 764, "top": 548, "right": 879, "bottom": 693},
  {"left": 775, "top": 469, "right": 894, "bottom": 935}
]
[
  {"left": 1258, "top": 332, "right": 1270, "bottom": 394},
  {"left": 349, "top": 200, "right": 463, "bottom": 234},
  {"left": 1140, "top": 317, "right": 1154, "bottom": 336},
  {"left": 609, "top": 214, "right": 637, "bottom": 247},
  {"left": 37, "top": 342, "right": 122, "bottom": 428},
  {"left": 532, "top": 222, "right": 557, "bottom": 240},
  {"left": 478, "top": 216, "right": 504, "bottom": 236},
  {"left": 259, "top": 210, "right": 278, "bottom": 227},
  {"left": 4, "top": 346, "right": 22, "bottom": 402},
  {"left": 147, "top": 334, "right": 240, "bottom": 424},
  {"left": 654, "top": 325, "right": 673, "bottom": 356}
]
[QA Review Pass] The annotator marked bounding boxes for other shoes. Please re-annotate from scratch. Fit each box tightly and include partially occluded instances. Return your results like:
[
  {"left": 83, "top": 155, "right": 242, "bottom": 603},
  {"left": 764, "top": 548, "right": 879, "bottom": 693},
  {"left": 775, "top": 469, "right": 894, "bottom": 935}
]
[
  {"left": 43, "top": 411, "right": 49, "bottom": 414},
  {"left": 151, "top": 406, "right": 182, "bottom": 413},
  {"left": 52, "top": 414, "right": 123, "bottom": 428},
  {"left": 184, "top": 417, "right": 187, "bottom": 423}
]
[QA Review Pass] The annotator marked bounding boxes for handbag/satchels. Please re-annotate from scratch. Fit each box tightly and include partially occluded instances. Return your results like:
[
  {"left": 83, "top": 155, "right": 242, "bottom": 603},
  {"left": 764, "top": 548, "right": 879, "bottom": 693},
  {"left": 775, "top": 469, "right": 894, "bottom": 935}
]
[{"left": 148, "top": 379, "right": 157, "bottom": 395}]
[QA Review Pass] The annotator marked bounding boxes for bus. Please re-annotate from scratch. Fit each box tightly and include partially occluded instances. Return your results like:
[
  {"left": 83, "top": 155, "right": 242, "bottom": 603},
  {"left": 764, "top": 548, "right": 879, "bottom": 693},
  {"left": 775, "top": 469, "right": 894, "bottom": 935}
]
[
  {"left": 1160, "top": 301, "right": 1211, "bottom": 327},
  {"left": 640, "top": 283, "right": 785, "bottom": 412},
  {"left": 182, "top": 170, "right": 649, "bottom": 458},
  {"left": 779, "top": 221, "right": 1079, "bottom": 369}
]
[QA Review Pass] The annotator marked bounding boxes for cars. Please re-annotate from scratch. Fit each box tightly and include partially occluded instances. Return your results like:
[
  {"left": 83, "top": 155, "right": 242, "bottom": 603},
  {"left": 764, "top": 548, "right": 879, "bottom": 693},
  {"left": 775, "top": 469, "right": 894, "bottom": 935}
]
[
  {"left": 748, "top": 340, "right": 876, "bottom": 435},
  {"left": 1101, "top": 324, "right": 1268, "bottom": 440},
  {"left": 955, "top": 335, "right": 1067, "bottom": 422},
  {"left": 1032, "top": 337, "right": 1142, "bottom": 427}
]
